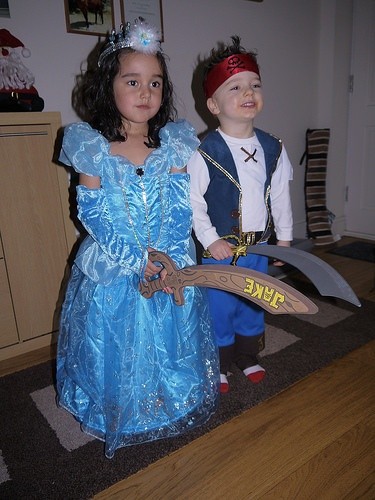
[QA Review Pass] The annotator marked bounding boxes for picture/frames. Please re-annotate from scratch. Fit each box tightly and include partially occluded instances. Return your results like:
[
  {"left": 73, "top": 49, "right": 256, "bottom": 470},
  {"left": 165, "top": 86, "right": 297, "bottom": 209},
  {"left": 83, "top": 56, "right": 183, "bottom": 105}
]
[
  {"left": 64, "top": 0, "right": 116, "bottom": 37},
  {"left": 120, "top": 0, "right": 165, "bottom": 43}
]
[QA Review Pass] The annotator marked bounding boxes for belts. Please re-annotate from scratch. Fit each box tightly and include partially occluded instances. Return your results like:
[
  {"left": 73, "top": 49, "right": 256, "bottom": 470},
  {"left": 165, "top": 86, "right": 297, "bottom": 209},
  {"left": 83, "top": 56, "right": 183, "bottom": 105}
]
[{"left": 239, "top": 232, "right": 264, "bottom": 245}]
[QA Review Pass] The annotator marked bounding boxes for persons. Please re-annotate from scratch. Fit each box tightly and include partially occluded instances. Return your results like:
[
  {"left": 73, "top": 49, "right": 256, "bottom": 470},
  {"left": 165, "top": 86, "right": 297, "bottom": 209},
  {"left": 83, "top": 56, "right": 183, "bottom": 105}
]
[
  {"left": 57, "top": 16, "right": 220, "bottom": 458},
  {"left": 187, "top": 35, "right": 294, "bottom": 395}
]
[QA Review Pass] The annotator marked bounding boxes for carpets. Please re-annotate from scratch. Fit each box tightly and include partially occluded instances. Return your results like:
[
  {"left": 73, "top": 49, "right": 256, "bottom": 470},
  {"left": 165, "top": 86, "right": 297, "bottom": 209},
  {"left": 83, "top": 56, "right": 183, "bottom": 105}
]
[{"left": 0, "top": 276, "right": 375, "bottom": 500}]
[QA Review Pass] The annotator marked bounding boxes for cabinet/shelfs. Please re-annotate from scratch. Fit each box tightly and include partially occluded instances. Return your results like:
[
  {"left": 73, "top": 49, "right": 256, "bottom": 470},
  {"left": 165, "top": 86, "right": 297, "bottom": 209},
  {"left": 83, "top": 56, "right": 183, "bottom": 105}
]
[{"left": 0, "top": 112, "right": 78, "bottom": 378}]
